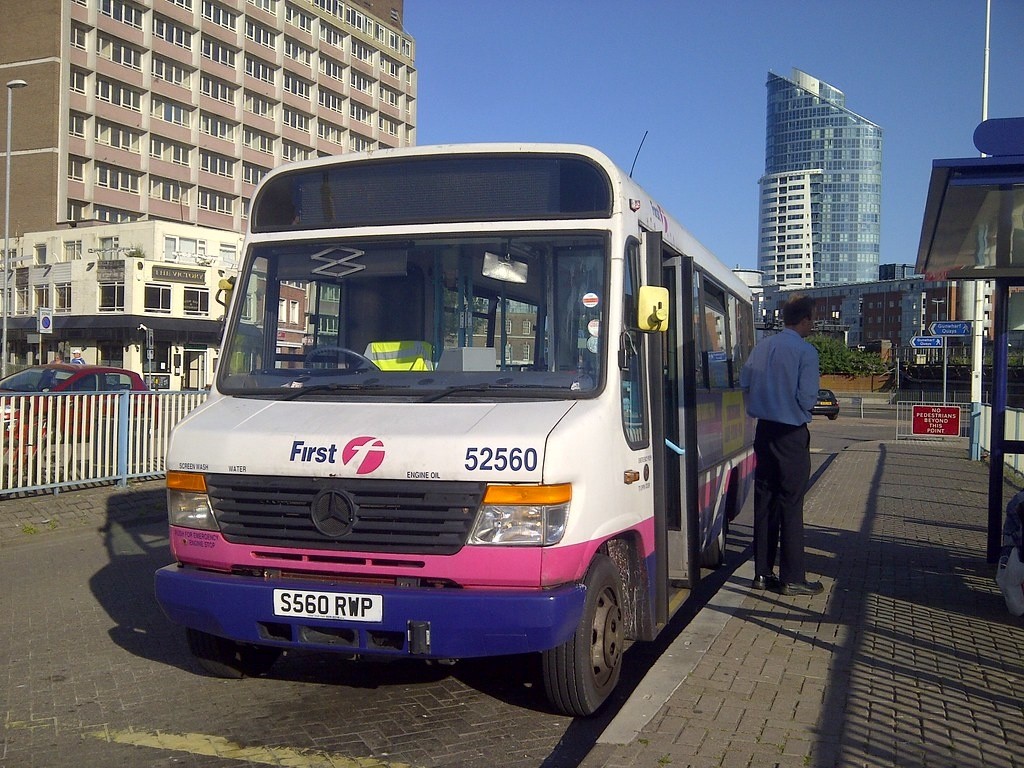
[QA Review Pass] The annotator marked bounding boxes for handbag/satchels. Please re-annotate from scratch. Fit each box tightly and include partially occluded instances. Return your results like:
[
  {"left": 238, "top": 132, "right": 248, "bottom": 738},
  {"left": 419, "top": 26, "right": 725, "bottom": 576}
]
[{"left": 995, "top": 547, "right": 1024, "bottom": 617}]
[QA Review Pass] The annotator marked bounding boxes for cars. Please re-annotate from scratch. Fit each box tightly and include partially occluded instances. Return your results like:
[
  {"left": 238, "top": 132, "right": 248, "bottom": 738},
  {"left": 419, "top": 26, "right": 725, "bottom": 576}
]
[
  {"left": 809, "top": 389, "right": 840, "bottom": 420},
  {"left": 0, "top": 364, "right": 159, "bottom": 448}
]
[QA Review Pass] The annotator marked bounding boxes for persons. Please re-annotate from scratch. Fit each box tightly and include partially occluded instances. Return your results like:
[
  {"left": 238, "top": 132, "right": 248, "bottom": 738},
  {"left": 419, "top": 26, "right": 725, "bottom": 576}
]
[
  {"left": 71, "top": 348, "right": 85, "bottom": 364},
  {"left": 739, "top": 294, "right": 825, "bottom": 596},
  {"left": 50, "top": 354, "right": 65, "bottom": 364}
]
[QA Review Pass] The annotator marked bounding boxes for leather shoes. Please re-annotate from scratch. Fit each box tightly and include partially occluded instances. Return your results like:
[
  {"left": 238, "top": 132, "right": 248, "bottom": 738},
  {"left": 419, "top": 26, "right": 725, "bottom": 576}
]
[
  {"left": 781, "top": 579, "right": 824, "bottom": 595},
  {"left": 752, "top": 571, "right": 780, "bottom": 590}
]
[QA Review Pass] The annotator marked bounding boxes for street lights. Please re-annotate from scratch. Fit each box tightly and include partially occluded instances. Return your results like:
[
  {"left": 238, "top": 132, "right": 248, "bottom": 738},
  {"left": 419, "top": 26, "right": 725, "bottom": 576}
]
[{"left": 2, "top": 77, "right": 28, "bottom": 374}]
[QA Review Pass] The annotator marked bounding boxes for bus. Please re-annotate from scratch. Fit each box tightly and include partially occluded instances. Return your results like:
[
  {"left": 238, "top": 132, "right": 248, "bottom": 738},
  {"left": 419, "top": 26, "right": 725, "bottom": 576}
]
[{"left": 152, "top": 141, "right": 757, "bottom": 716}]
